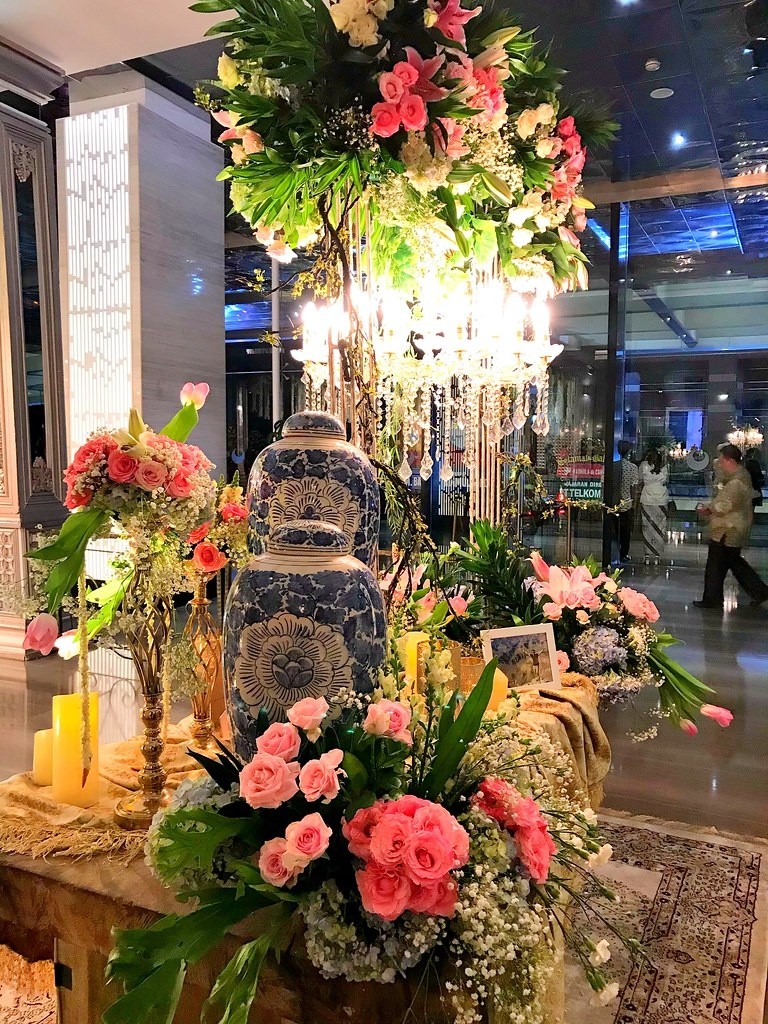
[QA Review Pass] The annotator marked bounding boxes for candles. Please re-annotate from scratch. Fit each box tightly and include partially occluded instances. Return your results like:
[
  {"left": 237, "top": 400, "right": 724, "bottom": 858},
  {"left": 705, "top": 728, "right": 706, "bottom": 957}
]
[
  {"left": 194, "top": 634, "right": 227, "bottom": 730},
  {"left": 33, "top": 728, "right": 53, "bottom": 785},
  {"left": 52, "top": 691, "right": 100, "bottom": 808}
]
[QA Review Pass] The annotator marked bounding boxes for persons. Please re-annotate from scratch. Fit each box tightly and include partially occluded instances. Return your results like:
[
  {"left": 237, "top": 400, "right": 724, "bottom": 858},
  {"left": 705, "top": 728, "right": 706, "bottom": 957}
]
[
  {"left": 601, "top": 441, "right": 638, "bottom": 562},
  {"left": 638, "top": 450, "right": 670, "bottom": 563},
  {"left": 694, "top": 445, "right": 768, "bottom": 608},
  {"left": 523, "top": 649, "right": 544, "bottom": 681}
]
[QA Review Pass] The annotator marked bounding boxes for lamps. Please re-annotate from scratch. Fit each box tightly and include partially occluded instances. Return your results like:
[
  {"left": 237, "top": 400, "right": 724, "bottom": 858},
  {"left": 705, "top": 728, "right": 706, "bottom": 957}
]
[
  {"left": 726, "top": 423, "right": 765, "bottom": 452},
  {"left": 669, "top": 443, "right": 689, "bottom": 460},
  {"left": 292, "top": 229, "right": 563, "bottom": 484}
]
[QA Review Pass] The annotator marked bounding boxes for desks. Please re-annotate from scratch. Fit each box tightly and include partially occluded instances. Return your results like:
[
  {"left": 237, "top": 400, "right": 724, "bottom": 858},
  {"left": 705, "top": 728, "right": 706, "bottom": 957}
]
[{"left": 0, "top": 684, "right": 609, "bottom": 1024}]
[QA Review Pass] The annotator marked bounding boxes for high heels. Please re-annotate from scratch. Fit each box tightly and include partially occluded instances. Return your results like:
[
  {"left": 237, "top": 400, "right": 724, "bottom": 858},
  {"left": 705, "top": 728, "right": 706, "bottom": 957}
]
[
  {"left": 653, "top": 556, "right": 660, "bottom": 565},
  {"left": 642, "top": 555, "right": 650, "bottom": 564}
]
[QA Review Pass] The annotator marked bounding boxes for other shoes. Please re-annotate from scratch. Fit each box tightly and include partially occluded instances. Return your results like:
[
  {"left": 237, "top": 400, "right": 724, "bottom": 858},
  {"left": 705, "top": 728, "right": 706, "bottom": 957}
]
[
  {"left": 620, "top": 555, "right": 632, "bottom": 563},
  {"left": 693, "top": 598, "right": 724, "bottom": 608},
  {"left": 750, "top": 586, "right": 768, "bottom": 606}
]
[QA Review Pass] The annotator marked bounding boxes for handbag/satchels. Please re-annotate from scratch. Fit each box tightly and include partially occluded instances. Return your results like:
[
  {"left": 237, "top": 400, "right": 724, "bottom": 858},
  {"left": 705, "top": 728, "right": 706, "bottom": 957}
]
[{"left": 668, "top": 501, "right": 676, "bottom": 513}]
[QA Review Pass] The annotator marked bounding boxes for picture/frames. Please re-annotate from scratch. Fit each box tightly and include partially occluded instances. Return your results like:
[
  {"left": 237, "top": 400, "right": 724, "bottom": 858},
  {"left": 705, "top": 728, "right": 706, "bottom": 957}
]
[{"left": 479, "top": 623, "right": 561, "bottom": 698}]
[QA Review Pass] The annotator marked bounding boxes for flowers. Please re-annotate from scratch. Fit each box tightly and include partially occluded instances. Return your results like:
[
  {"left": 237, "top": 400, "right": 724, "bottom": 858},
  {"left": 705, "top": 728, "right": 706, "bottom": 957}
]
[
  {"left": 373, "top": 454, "right": 735, "bottom": 747},
  {"left": 2, "top": 383, "right": 253, "bottom": 704},
  {"left": 186, "top": 0, "right": 621, "bottom": 343},
  {"left": 103, "top": 592, "right": 660, "bottom": 1024}
]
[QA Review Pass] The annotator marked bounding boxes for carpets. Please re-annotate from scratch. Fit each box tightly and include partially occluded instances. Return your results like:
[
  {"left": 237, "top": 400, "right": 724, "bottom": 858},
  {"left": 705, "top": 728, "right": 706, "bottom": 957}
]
[{"left": 560, "top": 806, "right": 768, "bottom": 1024}]
